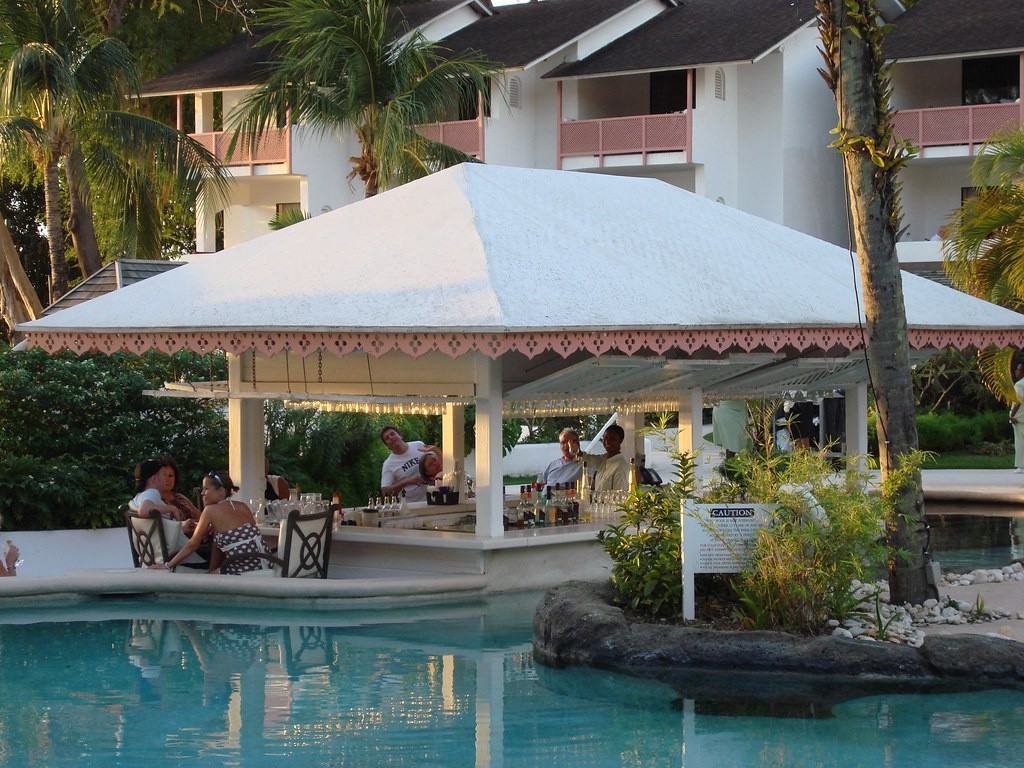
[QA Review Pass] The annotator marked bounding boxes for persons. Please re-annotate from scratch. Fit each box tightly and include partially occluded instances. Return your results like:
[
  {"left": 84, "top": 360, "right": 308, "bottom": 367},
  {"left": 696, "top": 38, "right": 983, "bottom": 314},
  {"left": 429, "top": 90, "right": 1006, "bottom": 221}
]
[
  {"left": 133, "top": 457, "right": 290, "bottom": 576},
  {"left": 1009, "top": 363, "right": 1024, "bottom": 474},
  {"left": 0, "top": 545, "right": 20, "bottom": 577},
  {"left": 541, "top": 425, "right": 630, "bottom": 505},
  {"left": 381, "top": 426, "right": 442, "bottom": 503}
]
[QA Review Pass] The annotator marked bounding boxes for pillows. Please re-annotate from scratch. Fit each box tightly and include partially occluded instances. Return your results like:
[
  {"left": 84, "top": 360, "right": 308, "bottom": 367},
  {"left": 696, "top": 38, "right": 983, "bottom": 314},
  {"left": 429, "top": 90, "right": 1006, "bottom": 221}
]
[
  {"left": 276, "top": 517, "right": 327, "bottom": 578},
  {"left": 131, "top": 517, "right": 181, "bottom": 567}
]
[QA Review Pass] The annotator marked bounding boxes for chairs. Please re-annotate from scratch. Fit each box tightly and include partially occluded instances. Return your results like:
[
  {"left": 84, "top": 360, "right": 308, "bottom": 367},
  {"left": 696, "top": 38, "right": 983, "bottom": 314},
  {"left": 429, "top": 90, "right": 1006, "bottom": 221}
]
[
  {"left": 117, "top": 505, "right": 211, "bottom": 570},
  {"left": 220, "top": 504, "right": 339, "bottom": 579},
  {"left": 193, "top": 487, "right": 204, "bottom": 513}
]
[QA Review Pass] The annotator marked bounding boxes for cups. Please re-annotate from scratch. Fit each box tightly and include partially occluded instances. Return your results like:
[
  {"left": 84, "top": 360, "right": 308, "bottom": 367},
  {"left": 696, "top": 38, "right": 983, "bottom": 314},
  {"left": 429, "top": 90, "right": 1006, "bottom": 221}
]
[{"left": 262, "top": 489, "right": 341, "bottom": 532}]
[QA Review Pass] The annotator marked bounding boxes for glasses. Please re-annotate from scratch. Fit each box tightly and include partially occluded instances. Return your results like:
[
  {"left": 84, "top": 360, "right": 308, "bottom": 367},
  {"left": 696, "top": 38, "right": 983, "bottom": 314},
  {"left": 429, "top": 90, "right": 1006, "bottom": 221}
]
[{"left": 208, "top": 470, "right": 224, "bottom": 486}]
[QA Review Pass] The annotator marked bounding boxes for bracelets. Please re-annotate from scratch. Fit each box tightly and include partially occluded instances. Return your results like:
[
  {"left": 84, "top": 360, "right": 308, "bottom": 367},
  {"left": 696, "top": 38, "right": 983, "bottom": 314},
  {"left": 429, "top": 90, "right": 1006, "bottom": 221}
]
[
  {"left": 7, "top": 567, "right": 16, "bottom": 569},
  {"left": 165, "top": 562, "right": 170, "bottom": 569}
]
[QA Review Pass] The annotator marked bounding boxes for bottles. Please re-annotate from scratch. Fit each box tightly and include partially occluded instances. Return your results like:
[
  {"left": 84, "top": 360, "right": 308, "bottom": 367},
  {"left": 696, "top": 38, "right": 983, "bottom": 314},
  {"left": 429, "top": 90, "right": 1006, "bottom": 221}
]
[
  {"left": 629, "top": 458, "right": 637, "bottom": 499},
  {"left": 516, "top": 461, "right": 591, "bottom": 529},
  {"left": 374, "top": 492, "right": 382, "bottom": 510},
  {"left": 399, "top": 488, "right": 410, "bottom": 515},
  {"left": 368, "top": 493, "right": 374, "bottom": 509},
  {"left": 503, "top": 486, "right": 509, "bottom": 531},
  {"left": 383, "top": 494, "right": 390, "bottom": 510},
  {"left": 390, "top": 491, "right": 398, "bottom": 509}
]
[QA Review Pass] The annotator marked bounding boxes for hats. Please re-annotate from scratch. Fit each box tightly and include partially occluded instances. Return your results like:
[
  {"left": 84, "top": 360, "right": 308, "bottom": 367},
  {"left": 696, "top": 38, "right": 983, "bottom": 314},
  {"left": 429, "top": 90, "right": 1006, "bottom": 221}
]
[{"left": 134, "top": 459, "right": 162, "bottom": 492}]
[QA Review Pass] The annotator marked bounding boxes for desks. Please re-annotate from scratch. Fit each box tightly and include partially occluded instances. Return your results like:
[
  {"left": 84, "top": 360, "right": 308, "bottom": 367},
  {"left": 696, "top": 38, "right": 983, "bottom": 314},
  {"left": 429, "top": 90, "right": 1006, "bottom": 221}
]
[{"left": 407, "top": 523, "right": 590, "bottom": 533}]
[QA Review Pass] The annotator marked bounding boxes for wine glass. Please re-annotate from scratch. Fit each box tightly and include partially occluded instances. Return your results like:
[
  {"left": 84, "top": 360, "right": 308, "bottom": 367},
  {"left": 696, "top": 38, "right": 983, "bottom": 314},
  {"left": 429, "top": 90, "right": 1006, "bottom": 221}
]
[
  {"left": 590, "top": 489, "right": 628, "bottom": 514},
  {"left": 2, "top": 540, "right": 24, "bottom": 567},
  {"left": 249, "top": 499, "right": 263, "bottom": 525}
]
